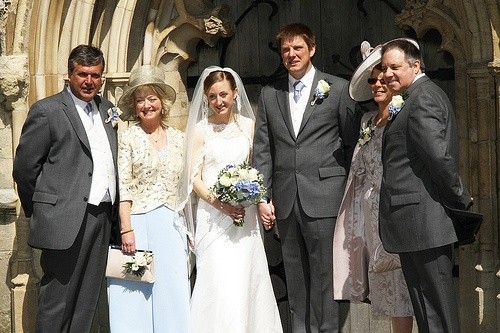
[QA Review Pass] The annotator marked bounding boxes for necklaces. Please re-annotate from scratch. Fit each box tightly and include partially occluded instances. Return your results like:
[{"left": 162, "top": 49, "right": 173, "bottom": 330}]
[{"left": 143, "top": 123, "right": 163, "bottom": 142}]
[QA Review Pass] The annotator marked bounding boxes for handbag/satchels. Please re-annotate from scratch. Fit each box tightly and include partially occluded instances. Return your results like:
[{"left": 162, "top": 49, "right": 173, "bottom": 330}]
[{"left": 105, "top": 246, "right": 154, "bottom": 284}]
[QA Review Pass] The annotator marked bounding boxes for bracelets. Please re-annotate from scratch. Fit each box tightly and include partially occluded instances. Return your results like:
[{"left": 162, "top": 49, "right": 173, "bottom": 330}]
[{"left": 120, "top": 228, "right": 134, "bottom": 235}]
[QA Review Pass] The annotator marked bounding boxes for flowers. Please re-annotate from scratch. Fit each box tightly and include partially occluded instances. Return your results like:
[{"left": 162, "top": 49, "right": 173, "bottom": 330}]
[
  {"left": 121, "top": 249, "right": 155, "bottom": 285},
  {"left": 104, "top": 103, "right": 123, "bottom": 128},
  {"left": 209, "top": 159, "right": 269, "bottom": 228},
  {"left": 385, "top": 91, "right": 409, "bottom": 121},
  {"left": 357, "top": 115, "right": 378, "bottom": 148},
  {"left": 310, "top": 78, "right": 333, "bottom": 106}
]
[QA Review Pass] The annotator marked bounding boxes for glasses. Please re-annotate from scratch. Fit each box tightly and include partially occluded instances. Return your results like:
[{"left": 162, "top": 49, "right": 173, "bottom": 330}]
[{"left": 367, "top": 77, "right": 385, "bottom": 84}]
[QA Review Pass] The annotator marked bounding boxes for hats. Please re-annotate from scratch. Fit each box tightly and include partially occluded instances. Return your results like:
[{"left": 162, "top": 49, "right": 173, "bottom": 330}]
[
  {"left": 117, "top": 65, "right": 177, "bottom": 122},
  {"left": 348, "top": 37, "right": 419, "bottom": 101}
]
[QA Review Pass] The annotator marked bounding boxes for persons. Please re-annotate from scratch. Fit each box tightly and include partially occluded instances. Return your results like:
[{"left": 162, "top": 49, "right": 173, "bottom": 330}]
[
  {"left": 378, "top": 39, "right": 484, "bottom": 333},
  {"left": 107, "top": 66, "right": 196, "bottom": 333},
  {"left": 13, "top": 45, "right": 119, "bottom": 333},
  {"left": 332, "top": 37, "right": 424, "bottom": 333},
  {"left": 252, "top": 23, "right": 364, "bottom": 333},
  {"left": 183, "top": 65, "right": 285, "bottom": 333}
]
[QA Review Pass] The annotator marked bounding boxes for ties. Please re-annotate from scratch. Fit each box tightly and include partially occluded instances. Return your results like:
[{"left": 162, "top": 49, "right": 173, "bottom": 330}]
[
  {"left": 293, "top": 81, "right": 304, "bottom": 103},
  {"left": 86, "top": 103, "right": 94, "bottom": 124}
]
